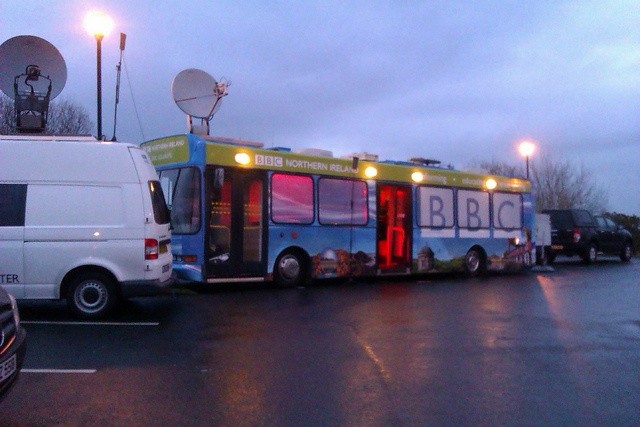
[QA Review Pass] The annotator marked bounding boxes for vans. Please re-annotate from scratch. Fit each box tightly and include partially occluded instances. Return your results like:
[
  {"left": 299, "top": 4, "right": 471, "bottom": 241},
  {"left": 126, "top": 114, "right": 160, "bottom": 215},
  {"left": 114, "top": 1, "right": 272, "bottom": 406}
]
[{"left": 0, "top": 134, "right": 175, "bottom": 321}]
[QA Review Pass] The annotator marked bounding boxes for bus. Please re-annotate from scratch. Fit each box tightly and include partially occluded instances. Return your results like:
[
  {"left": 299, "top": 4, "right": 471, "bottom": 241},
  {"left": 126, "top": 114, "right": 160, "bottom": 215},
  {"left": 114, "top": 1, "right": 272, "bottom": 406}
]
[{"left": 139, "top": 134, "right": 536, "bottom": 290}]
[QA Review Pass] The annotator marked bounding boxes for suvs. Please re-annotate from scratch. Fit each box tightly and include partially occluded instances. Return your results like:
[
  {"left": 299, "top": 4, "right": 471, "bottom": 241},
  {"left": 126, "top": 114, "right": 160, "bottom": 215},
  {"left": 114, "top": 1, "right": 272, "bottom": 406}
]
[{"left": 541, "top": 209, "right": 598, "bottom": 266}]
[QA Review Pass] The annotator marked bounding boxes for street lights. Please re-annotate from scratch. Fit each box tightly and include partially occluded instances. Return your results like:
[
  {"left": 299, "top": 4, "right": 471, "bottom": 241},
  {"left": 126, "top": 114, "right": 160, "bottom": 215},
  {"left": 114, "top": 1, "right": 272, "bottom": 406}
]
[
  {"left": 516, "top": 139, "right": 536, "bottom": 179},
  {"left": 87, "top": 9, "right": 114, "bottom": 139}
]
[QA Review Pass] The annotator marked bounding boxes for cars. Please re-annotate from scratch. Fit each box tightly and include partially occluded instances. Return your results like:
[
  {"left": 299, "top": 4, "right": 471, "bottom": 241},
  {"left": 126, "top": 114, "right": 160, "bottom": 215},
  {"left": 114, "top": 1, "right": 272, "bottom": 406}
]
[{"left": 595, "top": 216, "right": 634, "bottom": 262}]
